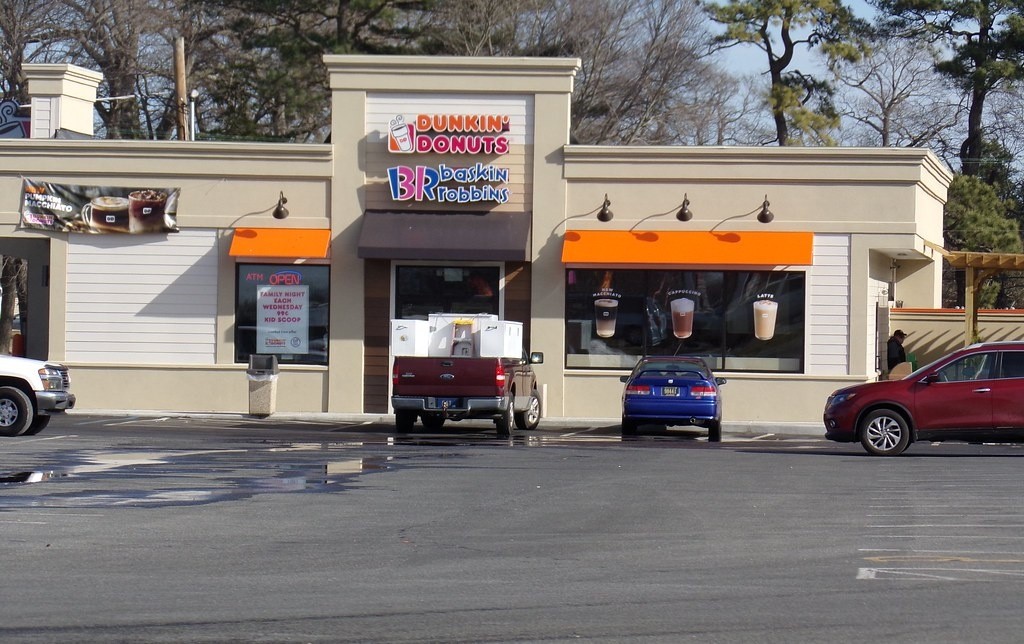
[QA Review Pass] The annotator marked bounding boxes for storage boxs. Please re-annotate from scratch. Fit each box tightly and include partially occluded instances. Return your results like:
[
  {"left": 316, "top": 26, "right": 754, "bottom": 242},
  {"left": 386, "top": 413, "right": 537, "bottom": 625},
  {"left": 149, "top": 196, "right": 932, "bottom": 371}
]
[
  {"left": 479, "top": 320, "right": 523, "bottom": 358},
  {"left": 428, "top": 314, "right": 498, "bottom": 358},
  {"left": 390, "top": 319, "right": 429, "bottom": 357}
]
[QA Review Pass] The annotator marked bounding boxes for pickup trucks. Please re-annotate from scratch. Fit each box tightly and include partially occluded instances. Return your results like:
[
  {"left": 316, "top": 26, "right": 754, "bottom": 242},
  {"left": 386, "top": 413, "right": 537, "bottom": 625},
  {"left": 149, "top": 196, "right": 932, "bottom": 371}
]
[{"left": 391, "top": 347, "right": 544, "bottom": 436}]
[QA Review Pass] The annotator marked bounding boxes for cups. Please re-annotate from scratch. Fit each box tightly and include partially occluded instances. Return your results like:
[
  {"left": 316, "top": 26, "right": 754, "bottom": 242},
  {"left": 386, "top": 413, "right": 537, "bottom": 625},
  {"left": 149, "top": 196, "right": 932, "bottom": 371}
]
[
  {"left": 754, "top": 301, "right": 778, "bottom": 340},
  {"left": 671, "top": 302, "right": 694, "bottom": 338},
  {"left": 595, "top": 301, "right": 618, "bottom": 337},
  {"left": 81, "top": 196, "right": 129, "bottom": 234},
  {"left": 129, "top": 193, "right": 167, "bottom": 234}
]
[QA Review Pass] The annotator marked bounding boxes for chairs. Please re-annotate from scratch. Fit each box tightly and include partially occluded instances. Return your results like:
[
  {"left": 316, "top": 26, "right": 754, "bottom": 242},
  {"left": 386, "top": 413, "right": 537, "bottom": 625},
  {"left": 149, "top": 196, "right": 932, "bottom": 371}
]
[
  {"left": 646, "top": 368, "right": 662, "bottom": 377},
  {"left": 974, "top": 354, "right": 992, "bottom": 379}
]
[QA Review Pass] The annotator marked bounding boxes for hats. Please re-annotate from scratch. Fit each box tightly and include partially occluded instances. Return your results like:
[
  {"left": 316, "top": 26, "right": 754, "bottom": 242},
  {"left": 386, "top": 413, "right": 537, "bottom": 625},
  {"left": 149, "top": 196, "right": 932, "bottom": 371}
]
[{"left": 894, "top": 329, "right": 907, "bottom": 336}]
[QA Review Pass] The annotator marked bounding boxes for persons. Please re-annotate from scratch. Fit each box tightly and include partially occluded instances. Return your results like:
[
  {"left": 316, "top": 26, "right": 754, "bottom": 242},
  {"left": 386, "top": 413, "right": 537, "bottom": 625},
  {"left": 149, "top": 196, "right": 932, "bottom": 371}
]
[{"left": 887, "top": 329, "right": 907, "bottom": 373}]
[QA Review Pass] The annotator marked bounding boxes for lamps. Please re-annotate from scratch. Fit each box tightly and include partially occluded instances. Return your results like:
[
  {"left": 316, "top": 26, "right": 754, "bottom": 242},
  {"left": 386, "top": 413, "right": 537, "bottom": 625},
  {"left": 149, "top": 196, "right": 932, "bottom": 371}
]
[
  {"left": 629, "top": 192, "right": 692, "bottom": 232},
  {"left": 710, "top": 193, "right": 774, "bottom": 232},
  {"left": 553, "top": 193, "right": 613, "bottom": 232},
  {"left": 890, "top": 265, "right": 901, "bottom": 269},
  {"left": 228, "top": 189, "right": 290, "bottom": 230}
]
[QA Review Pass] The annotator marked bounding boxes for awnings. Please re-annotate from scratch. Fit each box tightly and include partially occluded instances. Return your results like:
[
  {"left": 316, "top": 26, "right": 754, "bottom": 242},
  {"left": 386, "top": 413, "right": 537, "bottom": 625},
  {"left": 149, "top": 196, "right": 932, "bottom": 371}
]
[
  {"left": 229, "top": 229, "right": 331, "bottom": 258},
  {"left": 357, "top": 209, "right": 532, "bottom": 261},
  {"left": 561, "top": 230, "right": 814, "bottom": 266}
]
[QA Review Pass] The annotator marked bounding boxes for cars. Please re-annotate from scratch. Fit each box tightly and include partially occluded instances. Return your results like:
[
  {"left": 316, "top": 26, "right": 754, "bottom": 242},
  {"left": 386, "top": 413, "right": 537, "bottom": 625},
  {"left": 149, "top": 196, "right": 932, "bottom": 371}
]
[
  {"left": 619, "top": 355, "right": 727, "bottom": 441},
  {"left": 0, "top": 354, "right": 75, "bottom": 439}
]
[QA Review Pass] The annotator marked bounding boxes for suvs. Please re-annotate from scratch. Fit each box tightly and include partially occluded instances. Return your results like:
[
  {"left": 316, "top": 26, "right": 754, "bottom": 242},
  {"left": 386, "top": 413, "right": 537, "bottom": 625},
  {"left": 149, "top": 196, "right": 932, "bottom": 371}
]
[{"left": 823, "top": 341, "right": 1024, "bottom": 455}]
